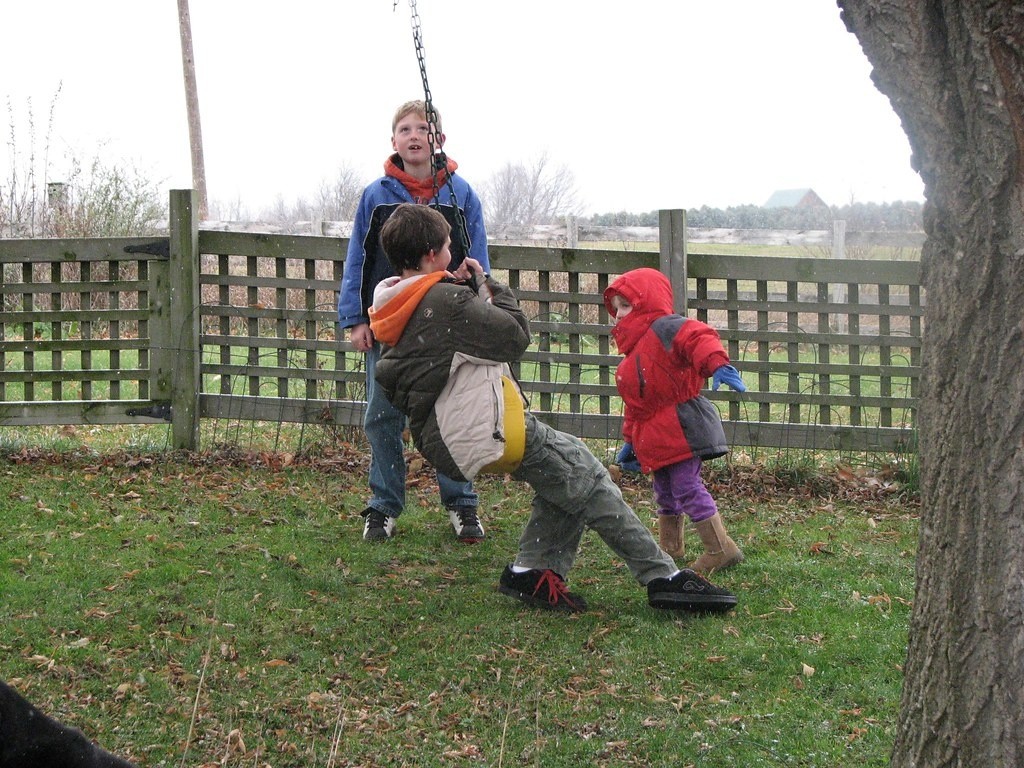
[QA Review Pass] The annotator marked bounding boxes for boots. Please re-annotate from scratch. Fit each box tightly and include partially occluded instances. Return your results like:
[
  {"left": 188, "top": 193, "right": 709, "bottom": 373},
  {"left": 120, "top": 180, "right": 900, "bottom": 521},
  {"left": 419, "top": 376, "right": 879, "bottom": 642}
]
[
  {"left": 688, "top": 512, "right": 742, "bottom": 575},
  {"left": 656, "top": 513, "right": 685, "bottom": 558}
]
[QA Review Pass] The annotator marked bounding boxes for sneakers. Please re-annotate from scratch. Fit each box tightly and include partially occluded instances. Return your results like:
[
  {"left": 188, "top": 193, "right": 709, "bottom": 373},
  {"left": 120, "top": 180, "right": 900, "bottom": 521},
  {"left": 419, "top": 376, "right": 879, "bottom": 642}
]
[
  {"left": 360, "top": 506, "right": 396, "bottom": 543},
  {"left": 498, "top": 562, "right": 586, "bottom": 613},
  {"left": 647, "top": 569, "right": 738, "bottom": 611},
  {"left": 448, "top": 506, "right": 485, "bottom": 544}
]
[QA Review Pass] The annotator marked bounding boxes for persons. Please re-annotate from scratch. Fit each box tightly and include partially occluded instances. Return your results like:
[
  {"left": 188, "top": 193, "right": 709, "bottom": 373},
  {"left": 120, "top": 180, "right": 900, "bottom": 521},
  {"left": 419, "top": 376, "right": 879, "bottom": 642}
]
[
  {"left": 602, "top": 268, "right": 746, "bottom": 577},
  {"left": 336, "top": 100, "right": 490, "bottom": 543},
  {"left": 366, "top": 202, "right": 739, "bottom": 611}
]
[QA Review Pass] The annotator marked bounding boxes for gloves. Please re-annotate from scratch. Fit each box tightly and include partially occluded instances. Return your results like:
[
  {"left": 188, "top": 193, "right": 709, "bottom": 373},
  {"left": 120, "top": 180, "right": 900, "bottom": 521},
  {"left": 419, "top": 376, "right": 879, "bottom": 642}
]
[
  {"left": 711, "top": 364, "right": 747, "bottom": 393},
  {"left": 617, "top": 443, "right": 640, "bottom": 471}
]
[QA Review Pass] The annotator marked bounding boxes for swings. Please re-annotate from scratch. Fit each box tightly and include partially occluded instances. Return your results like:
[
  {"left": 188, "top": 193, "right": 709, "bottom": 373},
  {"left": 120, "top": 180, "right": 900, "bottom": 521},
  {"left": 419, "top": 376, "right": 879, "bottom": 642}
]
[{"left": 394, "top": 0, "right": 524, "bottom": 477}]
